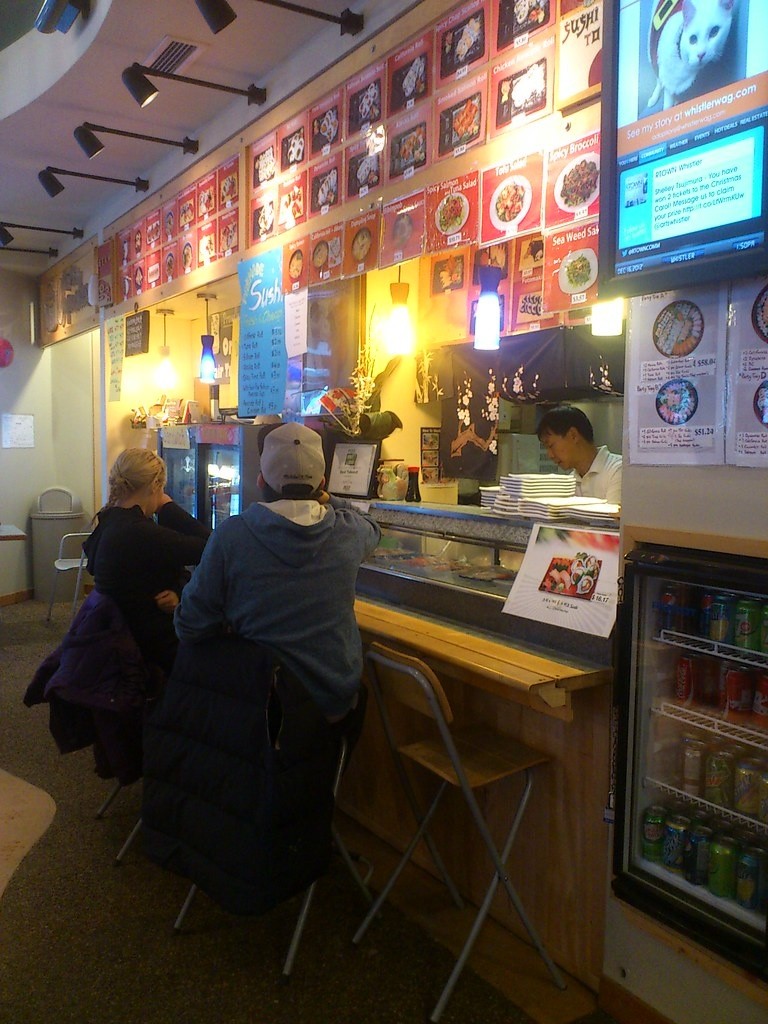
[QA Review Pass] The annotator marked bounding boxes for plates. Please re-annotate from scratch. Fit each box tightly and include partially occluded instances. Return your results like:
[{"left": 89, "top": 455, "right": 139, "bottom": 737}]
[
  {"left": 558, "top": 248, "right": 598, "bottom": 294},
  {"left": 134, "top": 211, "right": 193, "bottom": 290},
  {"left": 554, "top": 153, "right": 600, "bottom": 213},
  {"left": 490, "top": 175, "right": 532, "bottom": 231},
  {"left": 289, "top": 249, "right": 303, "bottom": 278},
  {"left": 753, "top": 380, "right": 768, "bottom": 428},
  {"left": 652, "top": 300, "right": 704, "bottom": 359},
  {"left": 311, "top": 240, "right": 329, "bottom": 268},
  {"left": 478, "top": 473, "right": 619, "bottom": 521},
  {"left": 751, "top": 284, "right": 768, "bottom": 344},
  {"left": 656, "top": 379, "right": 698, "bottom": 425},
  {"left": 326, "top": 236, "right": 342, "bottom": 268},
  {"left": 391, "top": 213, "right": 413, "bottom": 240},
  {"left": 352, "top": 227, "right": 372, "bottom": 260}
]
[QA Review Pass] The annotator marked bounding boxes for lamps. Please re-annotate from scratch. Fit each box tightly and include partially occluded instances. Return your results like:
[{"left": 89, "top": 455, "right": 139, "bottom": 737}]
[
  {"left": 74, "top": 122, "right": 198, "bottom": 159},
  {"left": 121, "top": 63, "right": 267, "bottom": 106},
  {"left": 474, "top": 247, "right": 502, "bottom": 352},
  {"left": 0, "top": 222, "right": 83, "bottom": 246},
  {"left": 198, "top": 294, "right": 217, "bottom": 384},
  {"left": 156, "top": 308, "right": 177, "bottom": 384},
  {"left": 388, "top": 265, "right": 415, "bottom": 354},
  {"left": 38, "top": 167, "right": 148, "bottom": 198},
  {"left": 194, "top": 0, "right": 364, "bottom": 36}
]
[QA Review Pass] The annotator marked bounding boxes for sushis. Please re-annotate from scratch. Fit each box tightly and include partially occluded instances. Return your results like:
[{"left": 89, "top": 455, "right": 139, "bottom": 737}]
[
  {"left": 659, "top": 383, "right": 697, "bottom": 423},
  {"left": 550, "top": 553, "right": 600, "bottom": 594},
  {"left": 654, "top": 302, "right": 702, "bottom": 355}
]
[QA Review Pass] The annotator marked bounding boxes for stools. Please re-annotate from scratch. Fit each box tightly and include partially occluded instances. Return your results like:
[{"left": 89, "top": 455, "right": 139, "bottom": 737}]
[
  {"left": 175, "top": 737, "right": 374, "bottom": 976},
  {"left": 349, "top": 642, "right": 567, "bottom": 1023}
]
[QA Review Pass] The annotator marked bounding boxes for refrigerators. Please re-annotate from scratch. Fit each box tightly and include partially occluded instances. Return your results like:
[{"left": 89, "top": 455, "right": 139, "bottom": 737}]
[
  {"left": 156, "top": 427, "right": 243, "bottom": 541},
  {"left": 611, "top": 544, "right": 768, "bottom": 984}
]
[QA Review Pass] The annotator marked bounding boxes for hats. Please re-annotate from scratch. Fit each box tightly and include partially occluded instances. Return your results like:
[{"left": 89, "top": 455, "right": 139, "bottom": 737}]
[{"left": 257, "top": 421, "right": 325, "bottom": 495}]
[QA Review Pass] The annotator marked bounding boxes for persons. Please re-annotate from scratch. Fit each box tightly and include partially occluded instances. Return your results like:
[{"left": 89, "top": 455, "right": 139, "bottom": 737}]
[
  {"left": 82, "top": 447, "right": 213, "bottom": 682},
  {"left": 173, "top": 421, "right": 383, "bottom": 739},
  {"left": 537, "top": 406, "right": 621, "bottom": 505}
]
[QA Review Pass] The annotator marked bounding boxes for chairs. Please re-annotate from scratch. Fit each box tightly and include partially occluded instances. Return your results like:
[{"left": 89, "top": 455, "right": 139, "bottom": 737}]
[{"left": 46, "top": 529, "right": 95, "bottom": 626}]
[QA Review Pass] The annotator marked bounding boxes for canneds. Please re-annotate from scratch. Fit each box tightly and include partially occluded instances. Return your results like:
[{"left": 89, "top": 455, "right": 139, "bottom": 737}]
[
  {"left": 661, "top": 584, "right": 768, "bottom": 731},
  {"left": 641, "top": 732, "right": 765, "bottom": 912}
]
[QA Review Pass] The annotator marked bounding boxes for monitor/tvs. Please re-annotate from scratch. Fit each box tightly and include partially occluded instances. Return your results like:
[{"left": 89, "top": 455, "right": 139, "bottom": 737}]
[{"left": 598, "top": 0, "right": 768, "bottom": 302}]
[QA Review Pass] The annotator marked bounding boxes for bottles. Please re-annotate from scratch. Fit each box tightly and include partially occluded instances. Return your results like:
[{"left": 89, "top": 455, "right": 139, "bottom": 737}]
[{"left": 435, "top": 192, "right": 470, "bottom": 236}]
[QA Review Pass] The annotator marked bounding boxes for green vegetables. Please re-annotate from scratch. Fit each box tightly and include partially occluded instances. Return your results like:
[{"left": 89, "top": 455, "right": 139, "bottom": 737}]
[{"left": 566, "top": 253, "right": 591, "bottom": 287}]
